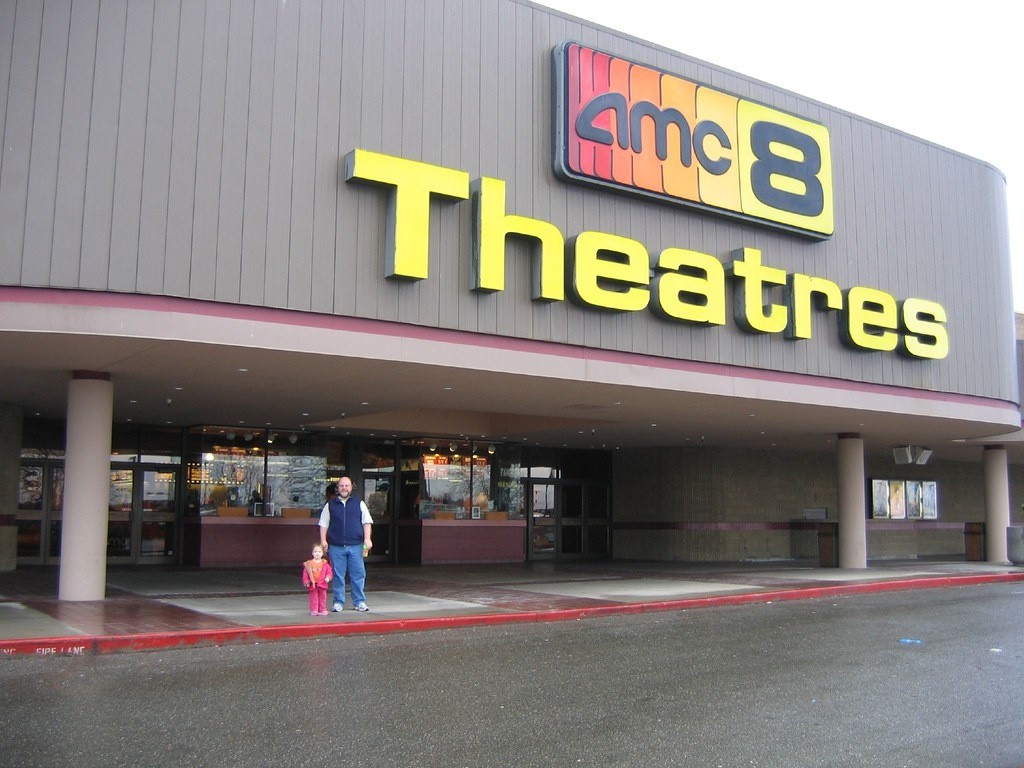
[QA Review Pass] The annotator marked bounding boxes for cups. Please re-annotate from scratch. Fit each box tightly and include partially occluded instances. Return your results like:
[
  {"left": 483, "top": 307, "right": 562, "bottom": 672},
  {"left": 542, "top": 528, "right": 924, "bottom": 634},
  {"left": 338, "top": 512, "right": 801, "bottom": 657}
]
[{"left": 362, "top": 544, "right": 368, "bottom": 557}]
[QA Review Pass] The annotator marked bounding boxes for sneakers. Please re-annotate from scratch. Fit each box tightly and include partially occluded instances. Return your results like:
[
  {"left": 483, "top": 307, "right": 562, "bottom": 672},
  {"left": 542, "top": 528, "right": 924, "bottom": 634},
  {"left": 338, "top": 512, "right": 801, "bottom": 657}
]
[
  {"left": 355, "top": 602, "right": 369, "bottom": 611},
  {"left": 332, "top": 602, "right": 342, "bottom": 612}
]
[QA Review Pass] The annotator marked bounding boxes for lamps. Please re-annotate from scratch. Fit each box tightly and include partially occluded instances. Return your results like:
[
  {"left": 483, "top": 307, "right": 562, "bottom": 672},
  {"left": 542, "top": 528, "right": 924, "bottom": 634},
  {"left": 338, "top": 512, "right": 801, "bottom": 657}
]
[
  {"left": 449, "top": 443, "right": 459, "bottom": 452},
  {"left": 227, "top": 428, "right": 297, "bottom": 443},
  {"left": 473, "top": 444, "right": 477, "bottom": 452},
  {"left": 430, "top": 443, "right": 437, "bottom": 451},
  {"left": 487, "top": 445, "right": 496, "bottom": 454}
]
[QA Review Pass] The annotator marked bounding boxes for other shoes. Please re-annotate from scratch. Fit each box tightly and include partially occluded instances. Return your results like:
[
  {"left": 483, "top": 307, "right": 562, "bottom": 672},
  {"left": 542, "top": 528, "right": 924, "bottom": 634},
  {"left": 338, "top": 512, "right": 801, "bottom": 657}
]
[
  {"left": 319, "top": 610, "right": 329, "bottom": 616},
  {"left": 311, "top": 610, "right": 320, "bottom": 616}
]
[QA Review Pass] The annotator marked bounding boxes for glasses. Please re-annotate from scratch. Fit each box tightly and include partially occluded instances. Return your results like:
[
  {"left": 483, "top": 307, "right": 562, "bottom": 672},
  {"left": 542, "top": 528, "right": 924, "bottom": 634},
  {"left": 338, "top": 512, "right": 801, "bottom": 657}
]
[{"left": 336, "top": 484, "right": 353, "bottom": 488}]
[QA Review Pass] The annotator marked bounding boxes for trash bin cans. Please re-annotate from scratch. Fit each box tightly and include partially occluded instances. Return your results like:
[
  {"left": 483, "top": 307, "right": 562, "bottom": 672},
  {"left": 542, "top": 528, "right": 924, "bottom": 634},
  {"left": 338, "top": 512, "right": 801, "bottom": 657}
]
[
  {"left": 964, "top": 521, "right": 985, "bottom": 561},
  {"left": 817, "top": 521, "right": 839, "bottom": 568}
]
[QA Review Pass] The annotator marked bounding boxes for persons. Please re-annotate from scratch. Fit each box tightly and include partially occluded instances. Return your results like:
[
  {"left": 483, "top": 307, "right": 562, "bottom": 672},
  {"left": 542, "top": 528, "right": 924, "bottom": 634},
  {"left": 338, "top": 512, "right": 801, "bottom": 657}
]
[
  {"left": 317, "top": 476, "right": 374, "bottom": 612},
  {"left": 368, "top": 483, "right": 389, "bottom": 555},
  {"left": 302, "top": 544, "right": 333, "bottom": 616}
]
